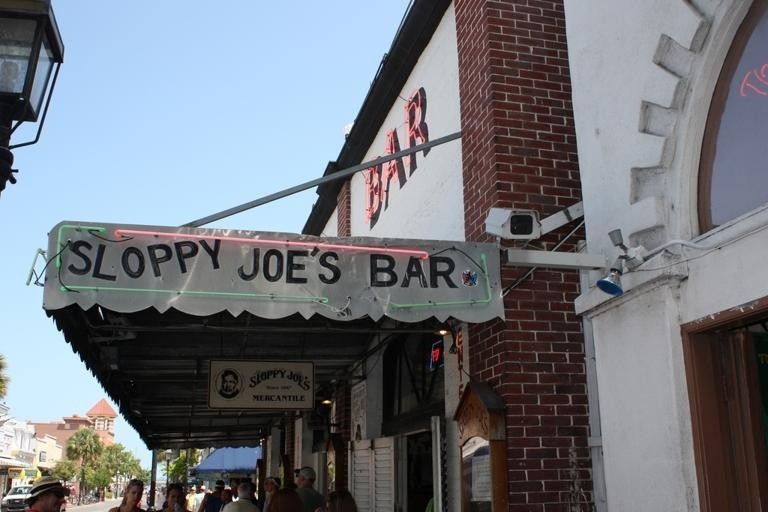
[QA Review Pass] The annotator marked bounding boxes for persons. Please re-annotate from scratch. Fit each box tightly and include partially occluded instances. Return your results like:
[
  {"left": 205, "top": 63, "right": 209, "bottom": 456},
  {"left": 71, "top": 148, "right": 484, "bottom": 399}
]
[
  {"left": 108, "top": 466, "right": 357, "bottom": 512},
  {"left": 219, "top": 370, "right": 240, "bottom": 399},
  {"left": 23, "top": 475, "right": 71, "bottom": 512}
]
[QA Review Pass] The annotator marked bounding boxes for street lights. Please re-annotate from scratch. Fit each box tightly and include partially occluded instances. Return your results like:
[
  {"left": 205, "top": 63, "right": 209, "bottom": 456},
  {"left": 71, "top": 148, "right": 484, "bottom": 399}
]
[
  {"left": 114, "top": 469, "right": 120, "bottom": 499},
  {"left": 164, "top": 449, "right": 173, "bottom": 497}
]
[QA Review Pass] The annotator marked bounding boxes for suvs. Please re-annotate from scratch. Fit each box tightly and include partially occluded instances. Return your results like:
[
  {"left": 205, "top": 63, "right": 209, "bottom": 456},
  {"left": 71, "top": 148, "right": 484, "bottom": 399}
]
[{"left": 1, "top": 484, "right": 34, "bottom": 511}]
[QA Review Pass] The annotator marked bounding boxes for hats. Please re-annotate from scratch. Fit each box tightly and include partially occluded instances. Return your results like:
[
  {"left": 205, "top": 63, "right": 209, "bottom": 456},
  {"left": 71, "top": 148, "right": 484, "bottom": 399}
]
[
  {"left": 24, "top": 476, "right": 70, "bottom": 505},
  {"left": 294, "top": 466, "right": 316, "bottom": 481}
]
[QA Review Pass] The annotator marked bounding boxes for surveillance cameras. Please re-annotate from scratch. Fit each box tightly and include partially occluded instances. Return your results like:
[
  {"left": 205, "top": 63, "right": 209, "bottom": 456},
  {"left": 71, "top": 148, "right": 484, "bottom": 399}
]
[{"left": 484, "top": 205, "right": 542, "bottom": 244}]
